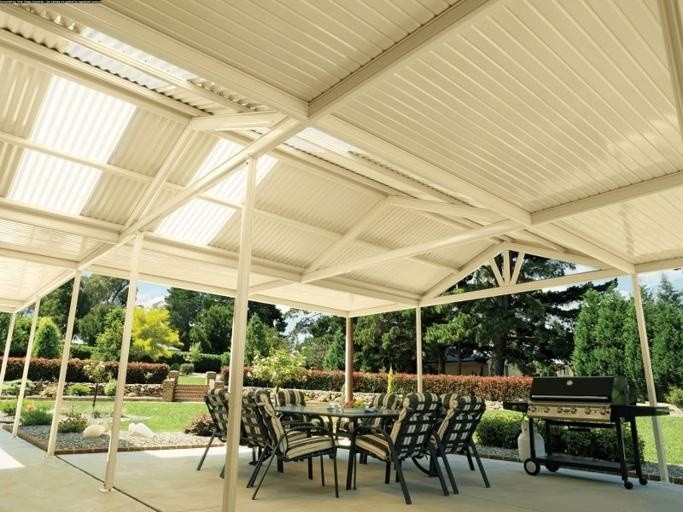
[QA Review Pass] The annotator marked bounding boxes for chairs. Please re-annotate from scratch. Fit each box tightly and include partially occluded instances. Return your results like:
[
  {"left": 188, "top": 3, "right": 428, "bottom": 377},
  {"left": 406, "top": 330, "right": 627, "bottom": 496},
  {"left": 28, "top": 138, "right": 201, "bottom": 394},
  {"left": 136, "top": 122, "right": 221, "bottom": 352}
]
[{"left": 195, "top": 385, "right": 491, "bottom": 504}]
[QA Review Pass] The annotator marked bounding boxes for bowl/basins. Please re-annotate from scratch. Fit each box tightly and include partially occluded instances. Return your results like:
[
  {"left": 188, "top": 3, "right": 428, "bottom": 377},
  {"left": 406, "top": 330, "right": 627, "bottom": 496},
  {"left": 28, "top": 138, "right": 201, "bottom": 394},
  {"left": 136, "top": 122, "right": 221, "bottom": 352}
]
[{"left": 327, "top": 407, "right": 336, "bottom": 412}]
